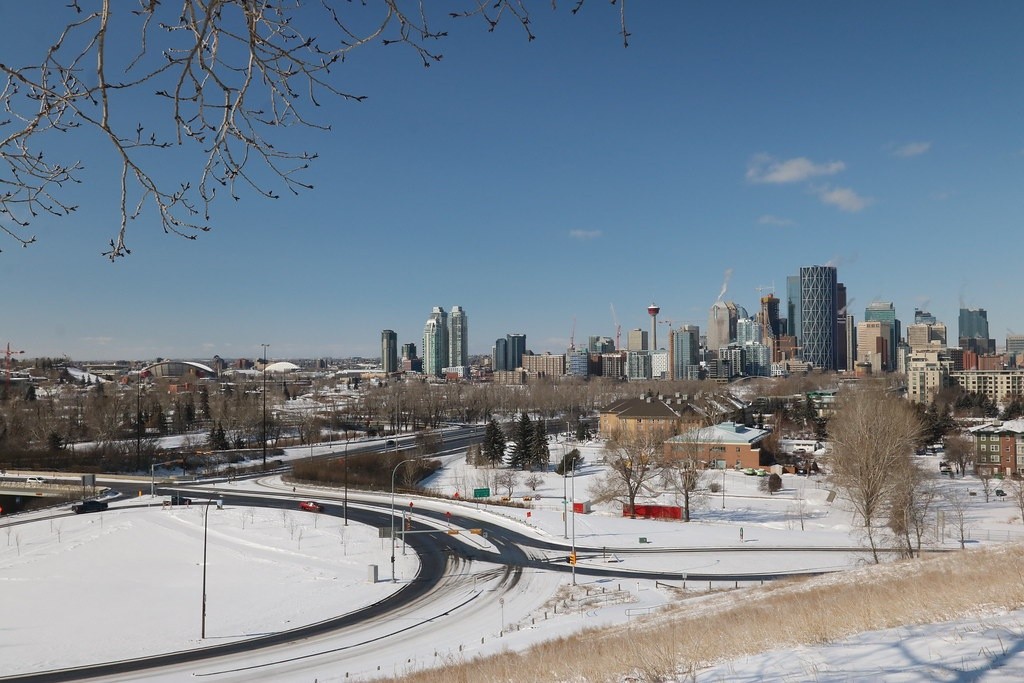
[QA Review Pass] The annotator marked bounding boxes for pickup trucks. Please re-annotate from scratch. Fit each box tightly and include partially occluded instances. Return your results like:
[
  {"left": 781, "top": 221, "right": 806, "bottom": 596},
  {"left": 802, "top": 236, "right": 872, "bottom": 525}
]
[
  {"left": 939, "top": 461, "right": 952, "bottom": 474},
  {"left": 162, "top": 495, "right": 191, "bottom": 506},
  {"left": 301, "top": 500, "right": 322, "bottom": 512},
  {"left": 70, "top": 500, "right": 108, "bottom": 514}
]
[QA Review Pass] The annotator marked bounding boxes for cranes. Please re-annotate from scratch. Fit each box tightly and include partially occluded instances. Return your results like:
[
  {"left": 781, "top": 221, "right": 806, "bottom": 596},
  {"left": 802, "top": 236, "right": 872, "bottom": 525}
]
[{"left": 0, "top": 342, "right": 25, "bottom": 406}]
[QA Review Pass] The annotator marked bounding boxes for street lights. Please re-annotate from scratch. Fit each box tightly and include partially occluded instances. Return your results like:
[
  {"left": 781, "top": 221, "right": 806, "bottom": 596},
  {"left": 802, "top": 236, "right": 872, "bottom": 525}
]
[
  {"left": 566, "top": 421, "right": 570, "bottom": 455},
  {"left": 389, "top": 459, "right": 417, "bottom": 584},
  {"left": 557, "top": 440, "right": 568, "bottom": 539},
  {"left": 259, "top": 343, "right": 272, "bottom": 470},
  {"left": 571, "top": 455, "right": 580, "bottom": 588}
]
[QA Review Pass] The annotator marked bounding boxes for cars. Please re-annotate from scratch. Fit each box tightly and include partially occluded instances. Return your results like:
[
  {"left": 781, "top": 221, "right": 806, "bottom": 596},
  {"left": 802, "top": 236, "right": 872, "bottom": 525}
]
[
  {"left": 996, "top": 490, "right": 1003, "bottom": 496},
  {"left": 743, "top": 467, "right": 766, "bottom": 476},
  {"left": 915, "top": 448, "right": 926, "bottom": 455},
  {"left": 27, "top": 476, "right": 47, "bottom": 485}
]
